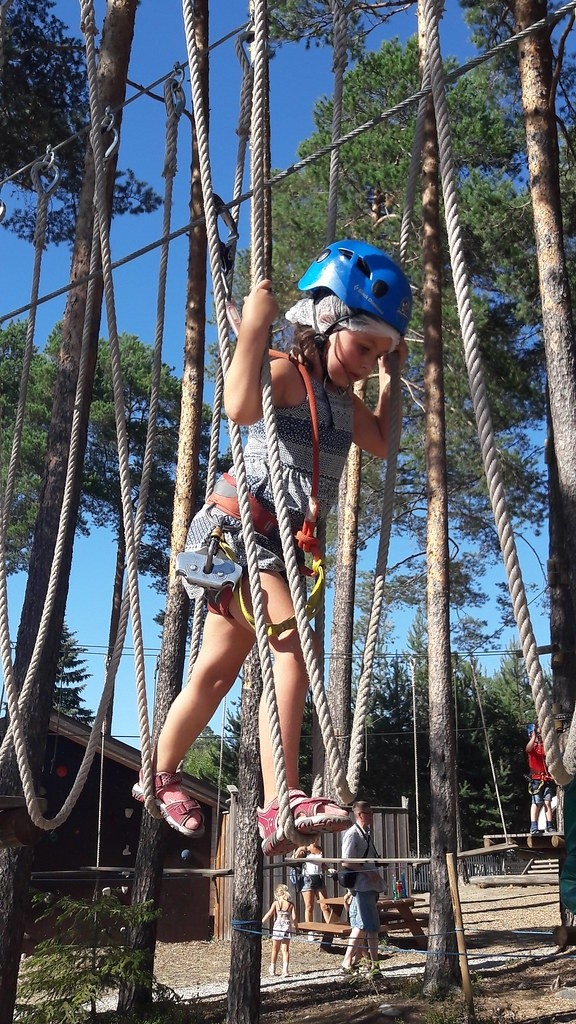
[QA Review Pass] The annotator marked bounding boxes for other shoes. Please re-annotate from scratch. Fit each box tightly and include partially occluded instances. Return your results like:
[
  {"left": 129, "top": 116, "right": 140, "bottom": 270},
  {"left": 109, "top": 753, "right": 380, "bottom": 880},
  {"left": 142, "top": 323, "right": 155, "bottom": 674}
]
[
  {"left": 282, "top": 973, "right": 293, "bottom": 977},
  {"left": 269, "top": 967, "right": 277, "bottom": 976}
]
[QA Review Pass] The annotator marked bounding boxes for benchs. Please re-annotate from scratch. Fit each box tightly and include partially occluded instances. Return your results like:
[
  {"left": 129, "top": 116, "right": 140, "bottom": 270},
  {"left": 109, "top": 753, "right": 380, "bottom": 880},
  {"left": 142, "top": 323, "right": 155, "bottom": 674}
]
[
  {"left": 379, "top": 911, "right": 429, "bottom": 922},
  {"left": 297, "top": 922, "right": 352, "bottom": 934}
]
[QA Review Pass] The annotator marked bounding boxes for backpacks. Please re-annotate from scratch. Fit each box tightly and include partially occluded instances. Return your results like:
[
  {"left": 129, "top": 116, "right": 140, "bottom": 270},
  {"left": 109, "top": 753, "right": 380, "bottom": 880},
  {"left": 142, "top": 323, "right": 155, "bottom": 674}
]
[{"left": 289, "top": 845, "right": 322, "bottom": 892}]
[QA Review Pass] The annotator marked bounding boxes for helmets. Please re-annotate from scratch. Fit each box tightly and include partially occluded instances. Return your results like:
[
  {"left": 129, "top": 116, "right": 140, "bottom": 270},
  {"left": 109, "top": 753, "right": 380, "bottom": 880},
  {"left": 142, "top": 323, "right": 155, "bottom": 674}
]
[
  {"left": 528, "top": 724, "right": 537, "bottom": 737},
  {"left": 297, "top": 238, "right": 412, "bottom": 336},
  {"left": 181, "top": 849, "right": 193, "bottom": 862}
]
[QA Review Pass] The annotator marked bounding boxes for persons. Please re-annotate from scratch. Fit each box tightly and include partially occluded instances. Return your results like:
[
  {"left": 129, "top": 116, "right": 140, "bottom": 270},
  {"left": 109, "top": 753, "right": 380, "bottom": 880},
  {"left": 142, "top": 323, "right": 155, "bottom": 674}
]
[
  {"left": 262, "top": 883, "right": 297, "bottom": 979},
  {"left": 133, "top": 240, "right": 412, "bottom": 854},
  {"left": 525, "top": 726, "right": 555, "bottom": 834},
  {"left": 292, "top": 837, "right": 336, "bottom": 941},
  {"left": 340, "top": 801, "right": 382, "bottom": 983}
]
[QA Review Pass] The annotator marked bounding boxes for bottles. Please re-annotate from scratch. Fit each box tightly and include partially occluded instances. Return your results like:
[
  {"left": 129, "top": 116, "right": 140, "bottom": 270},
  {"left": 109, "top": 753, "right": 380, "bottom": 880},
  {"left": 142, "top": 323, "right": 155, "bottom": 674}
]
[{"left": 391, "top": 873, "right": 407, "bottom": 900}]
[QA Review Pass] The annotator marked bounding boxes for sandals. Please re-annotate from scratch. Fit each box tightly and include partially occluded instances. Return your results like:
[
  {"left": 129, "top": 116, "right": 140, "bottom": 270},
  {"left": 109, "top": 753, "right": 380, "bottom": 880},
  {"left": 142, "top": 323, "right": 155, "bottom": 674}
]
[
  {"left": 368, "top": 966, "right": 383, "bottom": 979},
  {"left": 132, "top": 768, "right": 205, "bottom": 838},
  {"left": 530, "top": 828, "right": 539, "bottom": 834},
  {"left": 339, "top": 965, "right": 361, "bottom": 978},
  {"left": 548, "top": 826, "right": 556, "bottom": 832},
  {"left": 256, "top": 787, "right": 353, "bottom": 857}
]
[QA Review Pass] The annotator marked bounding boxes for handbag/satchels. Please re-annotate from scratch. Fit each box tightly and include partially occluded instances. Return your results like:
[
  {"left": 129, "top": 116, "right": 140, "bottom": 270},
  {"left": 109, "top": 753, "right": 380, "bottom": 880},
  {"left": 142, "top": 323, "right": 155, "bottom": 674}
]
[{"left": 337, "top": 866, "right": 359, "bottom": 888}]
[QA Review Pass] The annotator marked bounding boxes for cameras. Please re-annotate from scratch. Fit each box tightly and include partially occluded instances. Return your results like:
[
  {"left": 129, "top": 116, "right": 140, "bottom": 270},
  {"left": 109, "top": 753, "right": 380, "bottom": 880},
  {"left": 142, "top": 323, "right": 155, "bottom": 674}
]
[{"left": 376, "top": 855, "right": 389, "bottom": 867}]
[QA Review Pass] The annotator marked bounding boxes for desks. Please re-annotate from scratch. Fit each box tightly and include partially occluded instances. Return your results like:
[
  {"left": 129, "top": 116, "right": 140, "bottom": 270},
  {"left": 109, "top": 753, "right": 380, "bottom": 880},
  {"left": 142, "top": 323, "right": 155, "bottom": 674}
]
[{"left": 318, "top": 897, "right": 428, "bottom": 957}]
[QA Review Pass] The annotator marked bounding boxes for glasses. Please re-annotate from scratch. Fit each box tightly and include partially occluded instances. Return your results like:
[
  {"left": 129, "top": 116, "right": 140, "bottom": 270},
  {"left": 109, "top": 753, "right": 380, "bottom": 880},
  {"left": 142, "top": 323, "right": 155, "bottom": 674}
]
[{"left": 361, "top": 811, "right": 373, "bottom": 816}]
